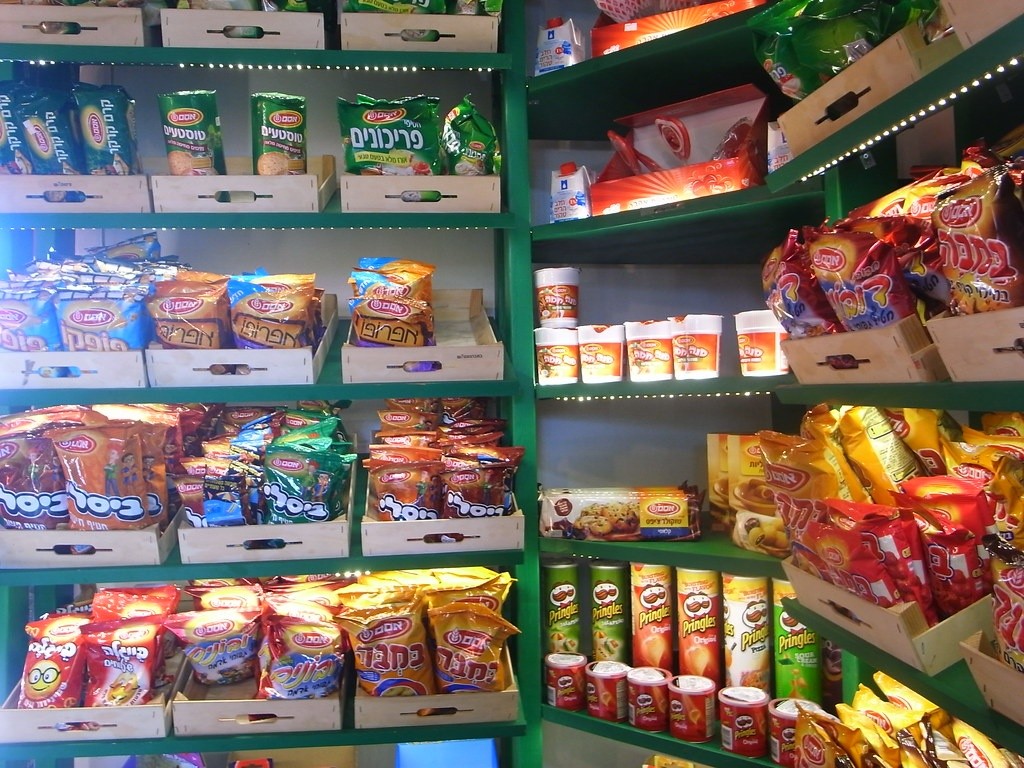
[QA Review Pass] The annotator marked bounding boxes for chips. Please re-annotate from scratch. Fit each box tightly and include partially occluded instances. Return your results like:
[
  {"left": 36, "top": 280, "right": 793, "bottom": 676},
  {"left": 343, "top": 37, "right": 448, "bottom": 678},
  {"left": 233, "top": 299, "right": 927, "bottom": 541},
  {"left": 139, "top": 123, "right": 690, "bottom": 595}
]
[
  {"left": 167, "top": 150, "right": 193, "bottom": 176},
  {"left": 643, "top": 637, "right": 709, "bottom": 677},
  {"left": 257, "top": 151, "right": 289, "bottom": 176}
]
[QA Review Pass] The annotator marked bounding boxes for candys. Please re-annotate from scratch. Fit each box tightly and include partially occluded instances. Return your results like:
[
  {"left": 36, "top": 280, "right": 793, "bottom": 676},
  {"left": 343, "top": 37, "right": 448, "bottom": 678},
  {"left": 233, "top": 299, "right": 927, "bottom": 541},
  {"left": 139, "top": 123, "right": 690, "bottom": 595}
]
[{"left": 608, "top": 116, "right": 750, "bottom": 175}]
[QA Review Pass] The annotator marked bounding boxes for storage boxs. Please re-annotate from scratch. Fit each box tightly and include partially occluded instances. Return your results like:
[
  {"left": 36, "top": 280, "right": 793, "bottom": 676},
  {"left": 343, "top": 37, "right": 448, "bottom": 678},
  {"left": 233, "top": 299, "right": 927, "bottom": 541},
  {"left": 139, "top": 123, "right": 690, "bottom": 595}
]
[
  {"left": 585, "top": 83, "right": 765, "bottom": 218},
  {"left": 777, "top": 26, "right": 962, "bottom": 160},
  {"left": 0, "top": 150, "right": 501, "bottom": 216},
  {"left": 779, "top": 551, "right": 1023, "bottom": 726},
  {"left": 0, "top": 0, "right": 501, "bottom": 53},
  {"left": 778, "top": 309, "right": 1024, "bottom": 384},
  {"left": 0, "top": 645, "right": 518, "bottom": 742},
  {"left": 5, "top": 289, "right": 506, "bottom": 390},
  {"left": 592, "top": 1, "right": 767, "bottom": 56},
  {"left": 0, "top": 432, "right": 526, "bottom": 568}
]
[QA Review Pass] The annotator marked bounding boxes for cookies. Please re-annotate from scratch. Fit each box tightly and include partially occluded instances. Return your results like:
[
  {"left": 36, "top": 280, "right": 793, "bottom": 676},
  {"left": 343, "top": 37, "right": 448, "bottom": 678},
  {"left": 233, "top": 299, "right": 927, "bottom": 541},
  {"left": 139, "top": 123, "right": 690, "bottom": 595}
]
[{"left": 573, "top": 501, "right": 644, "bottom": 540}]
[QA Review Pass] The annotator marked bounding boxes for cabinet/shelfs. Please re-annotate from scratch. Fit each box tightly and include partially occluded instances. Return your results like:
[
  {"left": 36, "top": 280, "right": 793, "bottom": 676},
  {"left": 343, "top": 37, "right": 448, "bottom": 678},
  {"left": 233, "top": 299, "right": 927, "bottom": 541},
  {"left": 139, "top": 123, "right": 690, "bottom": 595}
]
[
  {"left": 0, "top": 0, "right": 529, "bottom": 768},
  {"left": 526, "top": 0, "right": 1024, "bottom": 768}
]
